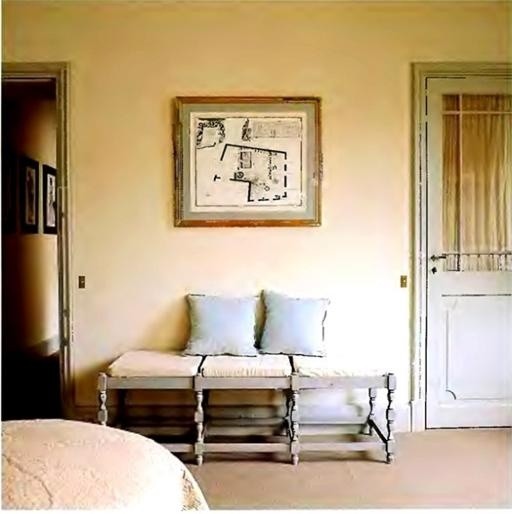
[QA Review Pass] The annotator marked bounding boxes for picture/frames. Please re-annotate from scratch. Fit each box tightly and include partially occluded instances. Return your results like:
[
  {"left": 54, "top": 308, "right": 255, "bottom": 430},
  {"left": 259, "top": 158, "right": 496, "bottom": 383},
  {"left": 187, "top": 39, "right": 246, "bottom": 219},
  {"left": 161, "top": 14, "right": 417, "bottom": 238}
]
[
  {"left": 21, "top": 152, "right": 57, "bottom": 236},
  {"left": 175, "top": 95, "right": 323, "bottom": 228}
]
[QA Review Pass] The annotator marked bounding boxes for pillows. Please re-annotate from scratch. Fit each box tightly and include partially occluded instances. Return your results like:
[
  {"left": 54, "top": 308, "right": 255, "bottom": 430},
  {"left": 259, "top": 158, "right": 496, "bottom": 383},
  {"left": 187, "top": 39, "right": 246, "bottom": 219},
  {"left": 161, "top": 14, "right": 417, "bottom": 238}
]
[{"left": 179, "top": 289, "right": 331, "bottom": 355}]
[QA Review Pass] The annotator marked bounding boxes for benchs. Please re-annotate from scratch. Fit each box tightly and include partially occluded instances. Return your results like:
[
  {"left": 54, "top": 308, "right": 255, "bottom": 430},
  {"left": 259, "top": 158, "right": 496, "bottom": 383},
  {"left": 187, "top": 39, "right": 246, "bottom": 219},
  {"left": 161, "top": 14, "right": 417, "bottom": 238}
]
[{"left": 96, "top": 348, "right": 398, "bottom": 464}]
